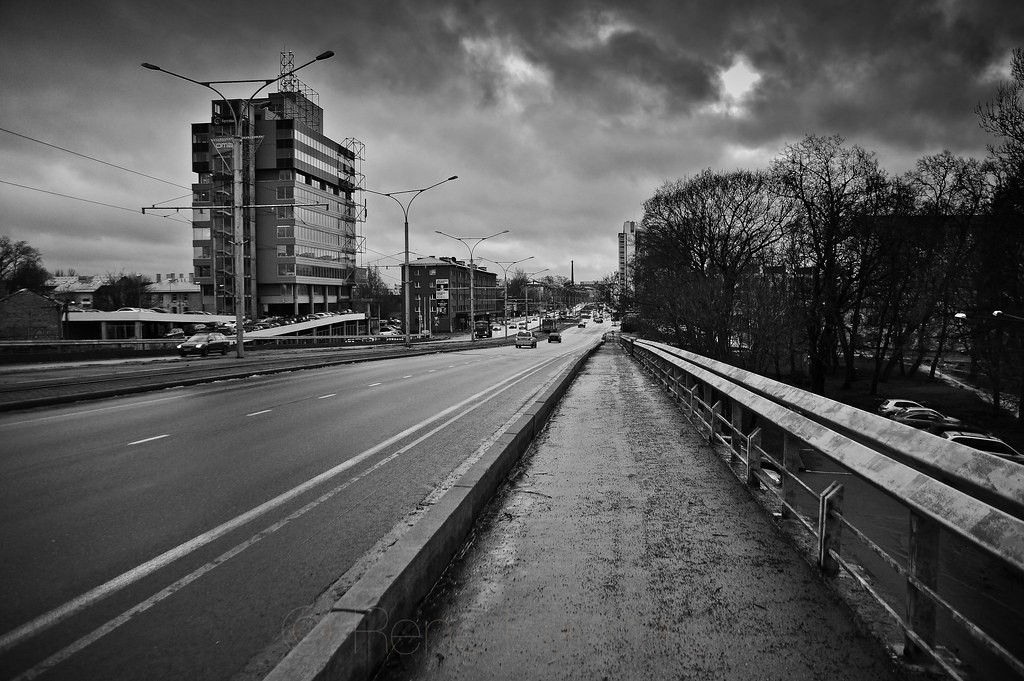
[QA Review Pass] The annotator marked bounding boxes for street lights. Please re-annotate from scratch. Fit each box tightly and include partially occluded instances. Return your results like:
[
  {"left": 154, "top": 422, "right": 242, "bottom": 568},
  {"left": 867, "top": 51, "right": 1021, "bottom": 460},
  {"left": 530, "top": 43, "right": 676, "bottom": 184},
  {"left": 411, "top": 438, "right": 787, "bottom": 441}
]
[
  {"left": 477, "top": 256, "right": 535, "bottom": 339},
  {"left": 359, "top": 175, "right": 459, "bottom": 347},
  {"left": 142, "top": 50, "right": 335, "bottom": 358},
  {"left": 434, "top": 230, "right": 511, "bottom": 343},
  {"left": 509, "top": 269, "right": 548, "bottom": 331}
]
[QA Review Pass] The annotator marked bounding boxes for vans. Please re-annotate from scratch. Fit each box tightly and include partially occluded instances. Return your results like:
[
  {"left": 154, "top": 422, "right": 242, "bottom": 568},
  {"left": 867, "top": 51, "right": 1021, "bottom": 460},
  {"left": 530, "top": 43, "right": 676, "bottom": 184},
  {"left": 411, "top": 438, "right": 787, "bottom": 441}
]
[
  {"left": 474, "top": 320, "right": 492, "bottom": 338},
  {"left": 519, "top": 321, "right": 528, "bottom": 329}
]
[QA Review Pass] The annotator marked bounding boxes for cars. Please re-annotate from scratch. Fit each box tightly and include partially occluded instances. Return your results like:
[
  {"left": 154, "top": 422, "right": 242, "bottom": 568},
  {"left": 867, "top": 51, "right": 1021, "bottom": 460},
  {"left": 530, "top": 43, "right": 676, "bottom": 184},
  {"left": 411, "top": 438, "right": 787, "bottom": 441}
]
[
  {"left": 533, "top": 316, "right": 537, "bottom": 321},
  {"left": 509, "top": 322, "right": 517, "bottom": 328},
  {"left": 419, "top": 330, "right": 433, "bottom": 339},
  {"left": 525, "top": 318, "right": 531, "bottom": 323},
  {"left": 179, "top": 333, "right": 231, "bottom": 357},
  {"left": 578, "top": 323, "right": 586, "bottom": 328},
  {"left": 545, "top": 303, "right": 593, "bottom": 322},
  {"left": 380, "top": 319, "right": 401, "bottom": 336},
  {"left": 594, "top": 317, "right": 603, "bottom": 324},
  {"left": 548, "top": 333, "right": 563, "bottom": 343},
  {"left": 184, "top": 308, "right": 353, "bottom": 337},
  {"left": 490, "top": 322, "right": 502, "bottom": 331},
  {"left": 67, "top": 307, "right": 171, "bottom": 313}
]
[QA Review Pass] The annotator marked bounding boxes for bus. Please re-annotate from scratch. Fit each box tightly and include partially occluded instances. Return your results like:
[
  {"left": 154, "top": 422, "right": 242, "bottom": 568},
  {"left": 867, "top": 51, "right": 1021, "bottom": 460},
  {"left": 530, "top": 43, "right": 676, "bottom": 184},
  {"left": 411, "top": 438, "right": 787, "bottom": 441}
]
[{"left": 543, "top": 319, "right": 558, "bottom": 332}]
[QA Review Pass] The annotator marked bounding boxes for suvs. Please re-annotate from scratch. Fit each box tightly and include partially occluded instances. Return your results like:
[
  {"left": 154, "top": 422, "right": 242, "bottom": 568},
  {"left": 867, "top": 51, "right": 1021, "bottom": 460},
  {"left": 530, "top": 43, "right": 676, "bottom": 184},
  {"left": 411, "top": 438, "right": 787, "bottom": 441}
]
[
  {"left": 161, "top": 328, "right": 186, "bottom": 338},
  {"left": 516, "top": 330, "right": 538, "bottom": 348},
  {"left": 880, "top": 400, "right": 1024, "bottom": 462}
]
